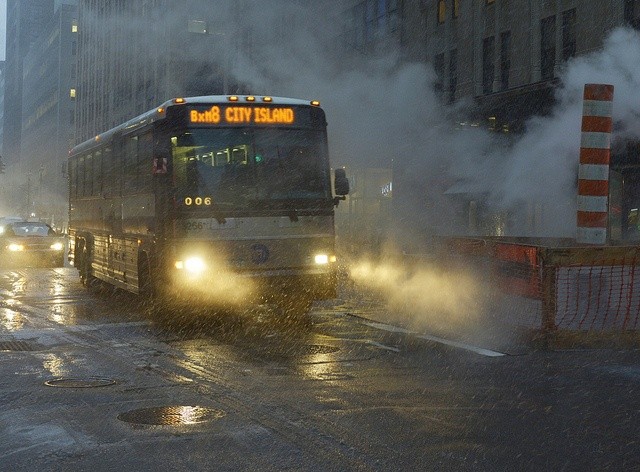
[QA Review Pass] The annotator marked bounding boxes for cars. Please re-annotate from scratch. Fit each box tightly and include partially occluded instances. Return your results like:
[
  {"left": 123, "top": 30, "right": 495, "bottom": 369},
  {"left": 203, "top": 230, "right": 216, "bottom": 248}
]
[
  {"left": 0, "top": 216, "right": 26, "bottom": 234},
  {"left": 0, "top": 220, "right": 64, "bottom": 268}
]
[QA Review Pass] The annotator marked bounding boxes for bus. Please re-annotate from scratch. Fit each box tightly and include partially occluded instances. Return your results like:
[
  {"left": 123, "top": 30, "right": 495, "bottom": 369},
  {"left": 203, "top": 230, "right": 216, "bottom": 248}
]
[{"left": 67, "top": 93, "right": 351, "bottom": 320}]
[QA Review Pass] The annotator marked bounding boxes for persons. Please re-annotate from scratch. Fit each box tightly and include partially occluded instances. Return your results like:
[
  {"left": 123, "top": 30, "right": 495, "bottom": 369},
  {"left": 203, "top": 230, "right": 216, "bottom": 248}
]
[{"left": 234, "top": 144, "right": 277, "bottom": 201}]
